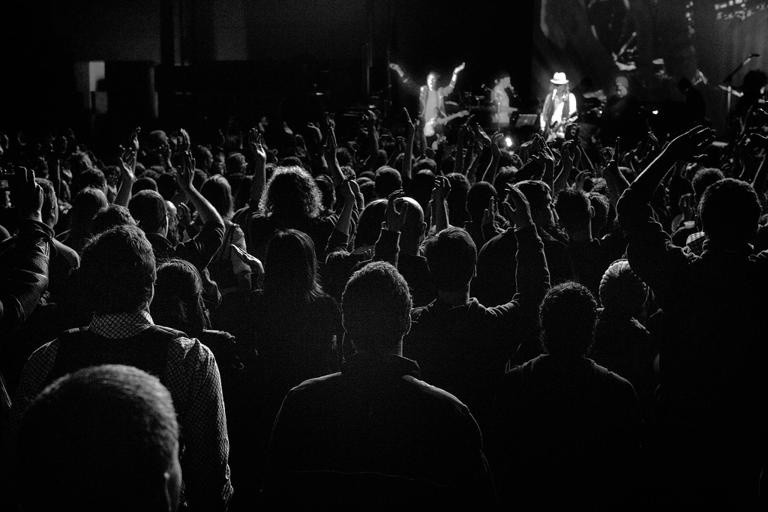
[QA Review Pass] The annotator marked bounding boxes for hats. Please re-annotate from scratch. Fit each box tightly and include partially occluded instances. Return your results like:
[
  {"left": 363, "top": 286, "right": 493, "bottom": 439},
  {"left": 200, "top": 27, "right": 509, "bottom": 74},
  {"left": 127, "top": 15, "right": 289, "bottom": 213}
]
[
  {"left": 550, "top": 71, "right": 569, "bottom": 85},
  {"left": 598, "top": 258, "right": 650, "bottom": 315}
]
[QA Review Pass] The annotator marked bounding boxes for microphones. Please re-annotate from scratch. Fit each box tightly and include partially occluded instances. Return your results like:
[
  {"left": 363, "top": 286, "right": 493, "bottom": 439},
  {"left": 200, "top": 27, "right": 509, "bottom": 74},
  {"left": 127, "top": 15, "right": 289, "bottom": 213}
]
[{"left": 551, "top": 88, "right": 558, "bottom": 101}]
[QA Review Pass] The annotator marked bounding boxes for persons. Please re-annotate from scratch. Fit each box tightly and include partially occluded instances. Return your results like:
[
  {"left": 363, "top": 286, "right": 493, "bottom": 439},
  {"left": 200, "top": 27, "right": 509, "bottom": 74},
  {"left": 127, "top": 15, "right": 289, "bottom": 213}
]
[
  {"left": 249, "top": 165, "right": 335, "bottom": 276},
  {"left": 0, "top": 106, "right": 391, "bottom": 190},
  {"left": 489, "top": 73, "right": 517, "bottom": 130},
  {"left": 388, "top": 198, "right": 437, "bottom": 307},
  {"left": 236, "top": 229, "right": 341, "bottom": 392},
  {"left": 494, "top": 283, "right": 643, "bottom": 512},
  {"left": 617, "top": 123, "right": 768, "bottom": 512},
  {"left": 402, "top": 184, "right": 550, "bottom": 408},
  {"left": 54, "top": 190, "right": 108, "bottom": 244},
  {"left": 388, "top": 61, "right": 466, "bottom": 138},
  {"left": 15, "top": 225, "right": 233, "bottom": 512},
  {"left": 618, "top": 116, "right": 768, "bottom": 242},
  {"left": 192, "top": 174, "right": 252, "bottom": 294},
  {"left": 464, "top": 181, "right": 506, "bottom": 245},
  {"left": 541, "top": 72, "right": 577, "bottom": 134},
  {"left": 9, "top": 367, "right": 183, "bottom": 512},
  {"left": 594, "top": 259, "right": 649, "bottom": 379},
  {"left": 34, "top": 179, "right": 81, "bottom": 285},
  {"left": 550, "top": 186, "right": 626, "bottom": 299},
  {"left": 129, "top": 151, "right": 215, "bottom": 277},
  {"left": 600, "top": 73, "right": 643, "bottom": 125},
  {"left": 480, "top": 179, "right": 563, "bottom": 304},
  {"left": 257, "top": 260, "right": 490, "bottom": 512},
  {"left": 330, "top": 173, "right": 387, "bottom": 293},
  {"left": 63, "top": 206, "right": 137, "bottom": 331},
  {"left": 148, "top": 259, "right": 252, "bottom": 452},
  {"left": 0, "top": 166, "right": 52, "bottom": 413},
  {"left": 395, "top": 122, "right": 614, "bottom": 194}
]
